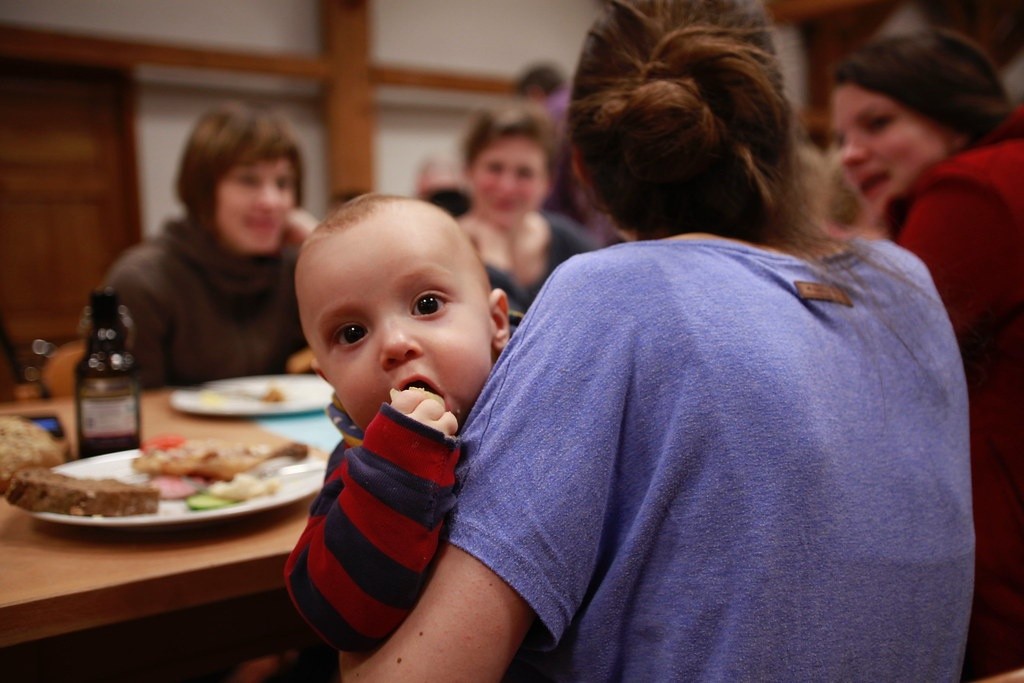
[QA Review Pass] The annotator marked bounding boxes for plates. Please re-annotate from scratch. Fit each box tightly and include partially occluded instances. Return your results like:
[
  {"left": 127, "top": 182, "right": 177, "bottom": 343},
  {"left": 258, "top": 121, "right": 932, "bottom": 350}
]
[
  {"left": 24, "top": 447, "right": 329, "bottom": 526},
  {"left": 169, "top": 373, "right": 343, "bottom": 415}
]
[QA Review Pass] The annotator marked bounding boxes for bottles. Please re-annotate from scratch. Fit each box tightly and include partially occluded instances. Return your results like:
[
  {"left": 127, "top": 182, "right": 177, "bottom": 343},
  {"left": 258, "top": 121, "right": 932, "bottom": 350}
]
[{"left": 75, "top": 287, "right": 141, "bottom": 461}]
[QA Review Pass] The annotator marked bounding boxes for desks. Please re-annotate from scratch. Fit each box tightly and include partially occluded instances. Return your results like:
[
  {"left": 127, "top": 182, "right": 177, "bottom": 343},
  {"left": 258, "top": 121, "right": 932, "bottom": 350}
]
[{"left": 1, "top": 361, "right": 346, "bottom": 649}]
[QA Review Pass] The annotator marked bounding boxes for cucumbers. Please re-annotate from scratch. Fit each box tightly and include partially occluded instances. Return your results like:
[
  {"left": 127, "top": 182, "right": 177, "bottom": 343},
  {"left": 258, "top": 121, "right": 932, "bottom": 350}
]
[{"left": 186, "top": 491, "right": 239, "bottom": 510}]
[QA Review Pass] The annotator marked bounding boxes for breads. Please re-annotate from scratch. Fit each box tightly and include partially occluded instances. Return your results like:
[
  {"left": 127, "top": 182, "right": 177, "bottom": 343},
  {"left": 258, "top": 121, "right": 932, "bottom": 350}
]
[
  {"left": 6, "top": 467, "right": 161, "bottom": 518},
  {"left": 0, "top": 414, "right": 66, "bottom": 493}
]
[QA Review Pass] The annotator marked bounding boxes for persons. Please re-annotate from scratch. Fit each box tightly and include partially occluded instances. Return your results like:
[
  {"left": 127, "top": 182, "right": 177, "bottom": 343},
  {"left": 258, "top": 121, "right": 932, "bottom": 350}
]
[
  {"left": 337, "top": 0, "right": 1024, "bottom": 683},
  {"left": 78, "top": 107, "right": 320, "bottom": 392},
  {"left": 283, "top": 193, "right": 526, "bottom": 655}
]
